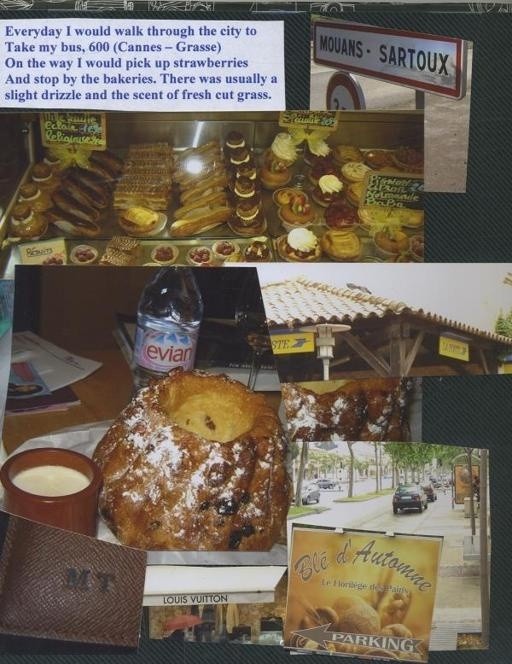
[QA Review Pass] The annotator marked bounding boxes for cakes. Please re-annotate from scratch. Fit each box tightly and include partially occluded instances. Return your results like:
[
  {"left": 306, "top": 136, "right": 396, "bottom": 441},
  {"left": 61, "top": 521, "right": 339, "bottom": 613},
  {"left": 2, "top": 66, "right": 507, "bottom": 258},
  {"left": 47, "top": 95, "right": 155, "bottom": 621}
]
[
  {"left": 281, "top": 376, "right": 418, "bottom": 441},
  {"left": 92, "top": 367, "right": 293, "bottom": 550},
  {"left": 9, "top": 132, "right": 423, "bottom": 265}
]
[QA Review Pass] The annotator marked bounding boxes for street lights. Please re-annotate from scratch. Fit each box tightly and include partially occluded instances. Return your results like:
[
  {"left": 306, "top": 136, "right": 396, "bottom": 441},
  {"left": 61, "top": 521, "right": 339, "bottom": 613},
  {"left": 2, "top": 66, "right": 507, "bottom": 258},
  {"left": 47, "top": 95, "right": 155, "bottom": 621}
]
[{"left": 299, "top": 323, "right": 353, "bottom": 380}]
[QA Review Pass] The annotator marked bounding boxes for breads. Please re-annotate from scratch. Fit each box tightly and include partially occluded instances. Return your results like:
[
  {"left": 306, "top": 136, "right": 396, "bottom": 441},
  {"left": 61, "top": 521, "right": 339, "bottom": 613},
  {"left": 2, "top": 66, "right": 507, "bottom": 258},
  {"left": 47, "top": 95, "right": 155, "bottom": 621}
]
[{"left": 299, "top": 585, "right": 412, "bottom": 657}]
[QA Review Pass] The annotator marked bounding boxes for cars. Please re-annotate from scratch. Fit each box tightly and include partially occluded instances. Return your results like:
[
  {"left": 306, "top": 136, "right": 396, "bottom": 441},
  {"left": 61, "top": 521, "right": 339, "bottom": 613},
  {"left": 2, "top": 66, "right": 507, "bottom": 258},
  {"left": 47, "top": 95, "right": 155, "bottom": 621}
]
[
  {"left": 314, "top": 474, "right": 338, "bottom": 491},
  {"left": 392, "top": 470, "right": 453, "bottom": 515},
  {"left": 292, "top": 482, "right": 322, "bottom": 505}
]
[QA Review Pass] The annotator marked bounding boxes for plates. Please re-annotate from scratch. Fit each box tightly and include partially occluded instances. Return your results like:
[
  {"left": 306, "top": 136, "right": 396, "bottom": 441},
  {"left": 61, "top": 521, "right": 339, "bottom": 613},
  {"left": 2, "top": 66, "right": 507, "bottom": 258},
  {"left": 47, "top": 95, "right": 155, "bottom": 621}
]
[{"left": 129, "top": 211, "right": 167, "bottom": 238}]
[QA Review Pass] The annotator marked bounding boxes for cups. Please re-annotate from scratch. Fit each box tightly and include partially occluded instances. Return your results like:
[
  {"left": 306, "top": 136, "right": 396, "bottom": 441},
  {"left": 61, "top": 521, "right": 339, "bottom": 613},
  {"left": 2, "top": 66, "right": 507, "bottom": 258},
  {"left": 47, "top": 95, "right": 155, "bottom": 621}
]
[{"left": 0, "top": 447, "right": 102, "bottom": 544}]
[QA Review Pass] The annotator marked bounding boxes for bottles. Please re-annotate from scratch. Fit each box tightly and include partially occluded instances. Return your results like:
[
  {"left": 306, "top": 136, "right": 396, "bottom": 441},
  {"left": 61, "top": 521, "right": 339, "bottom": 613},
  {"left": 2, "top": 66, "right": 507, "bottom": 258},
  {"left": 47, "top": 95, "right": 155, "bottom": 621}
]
[{"left": 130, "top": 266, "right": 206, "bottom": 394}]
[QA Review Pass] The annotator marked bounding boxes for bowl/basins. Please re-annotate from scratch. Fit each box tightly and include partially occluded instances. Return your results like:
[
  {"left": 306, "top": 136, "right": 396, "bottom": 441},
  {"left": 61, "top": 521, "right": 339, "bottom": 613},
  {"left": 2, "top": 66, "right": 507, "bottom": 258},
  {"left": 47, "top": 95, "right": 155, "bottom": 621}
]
[
  {"left": 69, "top": 243, "right": 273, "bottom": 268},
  {"left": 266, "top": 142, "right": 425, "bottom": 263}
]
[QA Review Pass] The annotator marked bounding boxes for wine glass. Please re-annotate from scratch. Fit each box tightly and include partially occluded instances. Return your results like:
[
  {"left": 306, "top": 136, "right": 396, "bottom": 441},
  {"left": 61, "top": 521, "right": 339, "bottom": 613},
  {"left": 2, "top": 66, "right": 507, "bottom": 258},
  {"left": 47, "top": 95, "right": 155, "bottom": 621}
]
[{"left": 233, "top": 270, "right": 274, "bottom": 392}]
